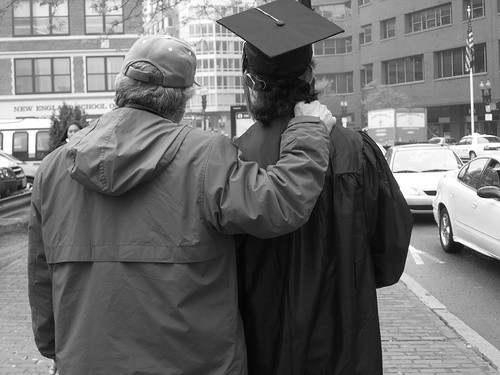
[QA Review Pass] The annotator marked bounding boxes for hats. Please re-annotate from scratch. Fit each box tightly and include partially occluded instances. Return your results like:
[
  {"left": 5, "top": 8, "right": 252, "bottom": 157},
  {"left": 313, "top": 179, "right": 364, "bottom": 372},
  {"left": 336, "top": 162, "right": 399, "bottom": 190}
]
[
  {"left": 216, "top": 0, "right": 346, "bottom": 78},
  {"left": 120, "top": 32, "right": 198, "bottom": 90}
]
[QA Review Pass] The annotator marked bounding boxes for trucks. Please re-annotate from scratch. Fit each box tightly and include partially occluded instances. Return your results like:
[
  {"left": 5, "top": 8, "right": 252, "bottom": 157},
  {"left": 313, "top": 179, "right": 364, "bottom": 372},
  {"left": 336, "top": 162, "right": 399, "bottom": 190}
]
[{"left": 361, "top": 105, "right": 428, "bottom": 148}]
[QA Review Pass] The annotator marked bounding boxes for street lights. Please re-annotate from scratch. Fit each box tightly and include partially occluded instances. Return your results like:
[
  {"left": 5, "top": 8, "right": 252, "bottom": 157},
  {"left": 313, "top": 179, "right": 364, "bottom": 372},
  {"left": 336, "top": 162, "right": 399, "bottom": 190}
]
[
  {"left": 339, "top": 97, "right": 348, "bottom": 129},
  {"left": 198, "top": 85, "right": 210, "bottom": 130},
  {"left": 218, "top": 118, "right": 226, "bottom": 135},
  {"left": 479, "top": 78, "right": 492, "bottom": 135}
]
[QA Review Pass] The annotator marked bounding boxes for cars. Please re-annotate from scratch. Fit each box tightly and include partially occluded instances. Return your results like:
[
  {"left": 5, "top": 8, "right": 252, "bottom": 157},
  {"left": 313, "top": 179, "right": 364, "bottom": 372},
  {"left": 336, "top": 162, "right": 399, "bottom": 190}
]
[
  {"left": 449, "top": 132, "right": 500, "bottom": 163},
  {"left": 385, "top": 142, "right": 464, "bottom": 214},
  {"left": 426, "top": 136, "right": 455, "bottom": 147},
  {"left": 0, "top": 151, "right": 28, "bottom": 200},
  {"left": 431, "top": 151, "right": 500, "bottom": 260}
]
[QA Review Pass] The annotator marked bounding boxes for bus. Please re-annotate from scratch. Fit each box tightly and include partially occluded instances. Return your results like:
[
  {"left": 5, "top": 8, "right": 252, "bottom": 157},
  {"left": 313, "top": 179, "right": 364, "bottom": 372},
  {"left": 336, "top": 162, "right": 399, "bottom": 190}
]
[{"left": 0, "top": 116, "right": 60, "bottom": 193}]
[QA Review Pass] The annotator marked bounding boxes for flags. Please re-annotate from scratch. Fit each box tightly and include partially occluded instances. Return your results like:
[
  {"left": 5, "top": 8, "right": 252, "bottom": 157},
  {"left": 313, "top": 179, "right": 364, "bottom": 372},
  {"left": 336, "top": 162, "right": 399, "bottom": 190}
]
[{"left": 464, "top": 11, "right": 475, "bottom": 72}]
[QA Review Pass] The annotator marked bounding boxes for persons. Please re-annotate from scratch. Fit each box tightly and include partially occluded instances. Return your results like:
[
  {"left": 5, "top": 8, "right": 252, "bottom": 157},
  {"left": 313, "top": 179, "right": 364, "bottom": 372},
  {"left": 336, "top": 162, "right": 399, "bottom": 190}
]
[
  {"left": 29, "top": 35, "right": 336, "bottom": 375},
  {"left": 55, "top": 123, "right": 83, "bottom": 147},
  {"left": 214, "top": 1, "right": 413, "bottom": 375}
]
